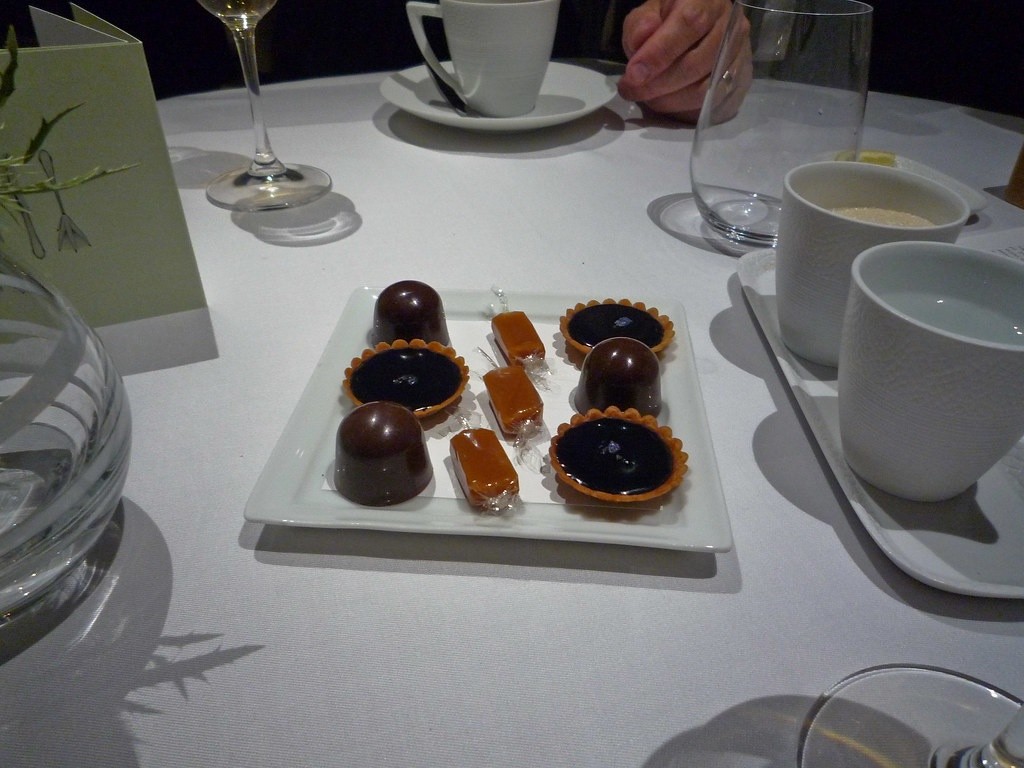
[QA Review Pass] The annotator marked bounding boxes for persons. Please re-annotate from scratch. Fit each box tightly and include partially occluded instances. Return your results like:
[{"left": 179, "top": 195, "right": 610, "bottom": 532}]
[{"left": 617, "top": 0, "right": 753, "bottom": 125}]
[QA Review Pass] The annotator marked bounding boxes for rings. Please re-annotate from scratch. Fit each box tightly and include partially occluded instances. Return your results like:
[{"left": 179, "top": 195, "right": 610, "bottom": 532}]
[{"left": 722, "top": 70, "right": 738, "bottom": 95}]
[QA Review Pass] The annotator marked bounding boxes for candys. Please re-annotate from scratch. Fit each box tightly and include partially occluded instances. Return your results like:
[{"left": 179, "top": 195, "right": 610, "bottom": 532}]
[{"left": 448, "top": 285, "right": 554, "bottom": 515}]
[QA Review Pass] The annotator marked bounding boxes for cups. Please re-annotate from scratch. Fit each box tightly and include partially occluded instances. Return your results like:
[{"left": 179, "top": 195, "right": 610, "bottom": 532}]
[
  {"left": 774, "top": 158, "right": 971, "bottom": 366},
  {"left": 689, "top": 0, "right": 874, "bottom": 248},
  {"left": 837, "top": 240, "right": 1024, "bottom": 500},
  {"left": 0, "top": 241, "right": 135, "bottom": 631},
  {"left": 406, "top": 0, "right": 561, "bottom": 117}
]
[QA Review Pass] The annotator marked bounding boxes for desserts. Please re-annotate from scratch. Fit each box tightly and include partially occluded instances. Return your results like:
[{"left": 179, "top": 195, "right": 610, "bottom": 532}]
[
  {"left": 334, "top": 280, "right": 469, "bottom": 507},
  {"left": 548, "top": 298, "right": 689, "bottom": 502}
]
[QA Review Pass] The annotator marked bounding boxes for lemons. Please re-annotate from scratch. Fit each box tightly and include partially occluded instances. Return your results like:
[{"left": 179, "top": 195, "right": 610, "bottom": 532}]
[{"left": 834, "top": 150, "right": 898, "bottom": 168}]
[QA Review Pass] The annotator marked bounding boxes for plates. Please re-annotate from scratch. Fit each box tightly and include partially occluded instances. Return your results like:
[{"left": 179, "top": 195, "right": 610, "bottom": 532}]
[
  {"left": 377, "top": 59, "right": 619, "bottom": 130},
  {"left": 241, "top": 285, "right": 733, "bottom": 553},
  {"left": 737, "top": 246, "right": 1024, "bottom": 598},
  {"left": 812, "top": 150, "right": 989, "bottom": 218}
]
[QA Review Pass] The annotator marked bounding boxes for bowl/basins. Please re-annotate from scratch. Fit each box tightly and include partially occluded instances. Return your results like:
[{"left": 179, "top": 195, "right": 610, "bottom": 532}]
[
  {"left": 342, "top": 338, "right": 470, "bottom": 417},
  {"left": 549, "top": 406, "right": 690, "bottom": 502},
  {"left": 559, "top": 297, "right": 676, "bottom": 356}
]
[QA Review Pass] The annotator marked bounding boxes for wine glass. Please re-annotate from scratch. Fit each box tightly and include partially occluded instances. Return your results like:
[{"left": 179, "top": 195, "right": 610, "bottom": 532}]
[
  {"left": 198, "top": 0, "right": 332, "bottom": 212},
  {"left": 798, "top": 661, "right": 1024, "bottom": 768}
]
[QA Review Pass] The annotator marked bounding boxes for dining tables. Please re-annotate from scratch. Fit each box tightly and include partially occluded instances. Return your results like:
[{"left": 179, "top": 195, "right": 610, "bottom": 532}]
[{"left": 1, "top": 55, "right": 1024, "bottom": 768}]
